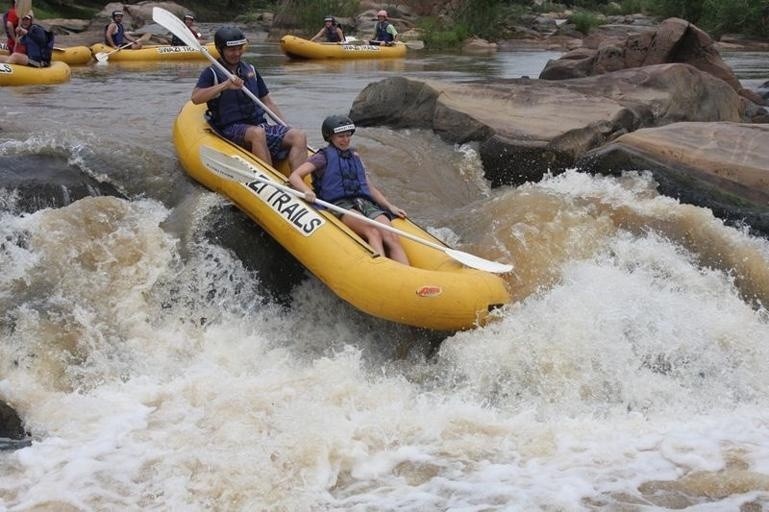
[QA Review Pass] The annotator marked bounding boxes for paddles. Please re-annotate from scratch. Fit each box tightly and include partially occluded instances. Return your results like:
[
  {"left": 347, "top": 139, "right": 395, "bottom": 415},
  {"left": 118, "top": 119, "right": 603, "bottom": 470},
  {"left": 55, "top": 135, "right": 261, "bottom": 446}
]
[
  {"left": 152, "top": 7, "right": 318, "bottom": 154},
  {"left": 199, "top": 146, "right": 513, "bottom": 273},
  {"left": 13, "top": 0, "right": 31, "bottom": 52},
  {"left": 95, "top": 33, "right": 151, "bottom": 62},
  {"left": 371, "top": 40, "right": 424, "bottom": 50}
]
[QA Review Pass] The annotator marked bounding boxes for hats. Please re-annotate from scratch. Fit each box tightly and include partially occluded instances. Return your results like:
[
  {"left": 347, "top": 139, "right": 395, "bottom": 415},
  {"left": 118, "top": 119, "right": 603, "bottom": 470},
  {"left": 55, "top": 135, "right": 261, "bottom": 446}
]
[
  {"left": 112, "top": 10, "right": 124, "bottom": 16},
  {"left": 22, "top": 15, "right": 32, "bottom": 20},
  {"left": 323, "top": 16, "right": 337, "bottom": 22},
  {"left": 183, "top": 14, "right": 194, "bottom": 22}
]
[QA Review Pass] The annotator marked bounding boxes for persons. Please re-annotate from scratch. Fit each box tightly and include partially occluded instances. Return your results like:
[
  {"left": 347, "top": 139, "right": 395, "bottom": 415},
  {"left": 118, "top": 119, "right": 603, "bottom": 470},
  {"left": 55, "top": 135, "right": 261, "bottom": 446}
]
[
  {"left": 103, "top": 10, "right": 142, "bottom": 50},
  {"left": 5, "top": 9, "right": 50, "bottom": 68},
  {"left": 2, "top": 0, "right": 19, "bottom": 55},
  {"left": 190, "top": 25, "right": 308, "bottom": 176},
  {"left": 288, "top": 113, "right": 412, "bottom": 266},
  {"left": 309, "top": 16, "right": 345, "bottom": 44},
  {"left": 367, "top": 10, "right": 399, "bottom": 48},
  {"left": 170, "top": 13, "right": 202, "bottom": 46}
]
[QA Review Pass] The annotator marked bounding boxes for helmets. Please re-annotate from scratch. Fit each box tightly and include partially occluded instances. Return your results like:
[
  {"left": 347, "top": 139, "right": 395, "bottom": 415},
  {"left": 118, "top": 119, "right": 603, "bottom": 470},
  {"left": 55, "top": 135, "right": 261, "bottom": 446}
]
[
  {"left": 377, "top": 10, "right": 388, "bottom": 16},
  {"left": 214, "top": 27, "right": 248, "bottom": 50},
  {"left": 322, "top": 114, "right": 356, "bottom": 142}
]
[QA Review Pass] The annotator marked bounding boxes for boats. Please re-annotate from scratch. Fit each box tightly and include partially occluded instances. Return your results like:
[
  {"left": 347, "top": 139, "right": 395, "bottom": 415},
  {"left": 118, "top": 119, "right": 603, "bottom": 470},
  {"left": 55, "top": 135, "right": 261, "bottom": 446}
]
[
  {"left": 173, "top": 100, "right": 509, "bottom": 330},
  {"left": 280, "top": 35, "right": 407, "bottom": 59},
  {"left": 0, "top": 43, "right": 220, "bottom": 86}
]
